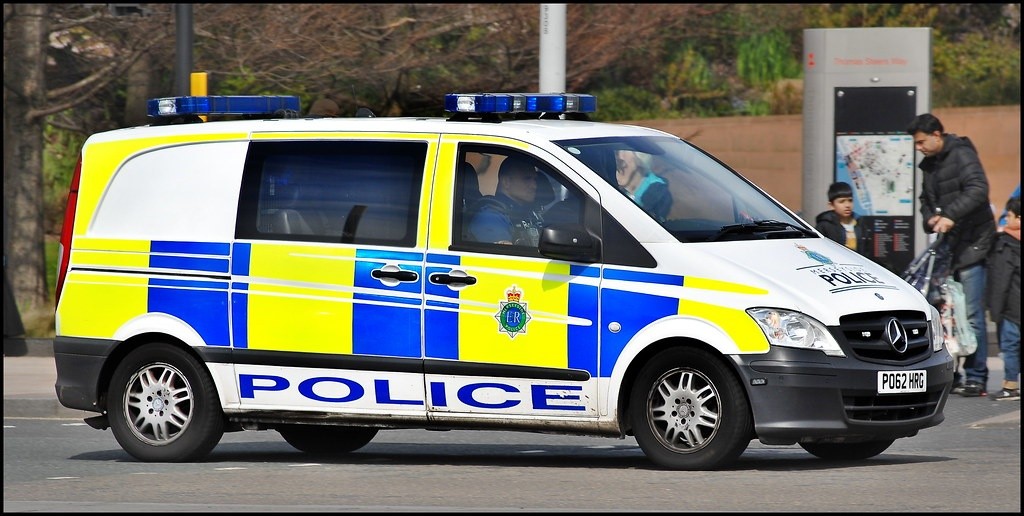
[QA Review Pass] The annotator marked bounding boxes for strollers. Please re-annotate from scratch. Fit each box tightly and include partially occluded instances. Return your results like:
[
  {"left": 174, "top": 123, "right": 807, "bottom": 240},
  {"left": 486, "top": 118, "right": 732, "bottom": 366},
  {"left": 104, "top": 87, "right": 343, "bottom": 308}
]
[{"left": 900, "top": 230, "right": 952, "bottom": 315}]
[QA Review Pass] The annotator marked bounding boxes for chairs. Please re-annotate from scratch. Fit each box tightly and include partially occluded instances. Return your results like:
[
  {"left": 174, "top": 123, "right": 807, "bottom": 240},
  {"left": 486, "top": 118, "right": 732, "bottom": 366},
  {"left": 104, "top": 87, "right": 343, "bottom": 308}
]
[
  {"left": 535, "top": 173, "right": 554, "bottom": 221},
  {"left": 462, "top": 162, "right": 482, "bottom": 244}
]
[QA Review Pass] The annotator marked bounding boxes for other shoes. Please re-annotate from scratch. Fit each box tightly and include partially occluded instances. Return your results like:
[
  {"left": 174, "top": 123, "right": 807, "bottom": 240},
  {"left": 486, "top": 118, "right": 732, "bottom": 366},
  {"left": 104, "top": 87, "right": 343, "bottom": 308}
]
[{"left": 997, "top": 351, "right": 1004, "bottom": 360}]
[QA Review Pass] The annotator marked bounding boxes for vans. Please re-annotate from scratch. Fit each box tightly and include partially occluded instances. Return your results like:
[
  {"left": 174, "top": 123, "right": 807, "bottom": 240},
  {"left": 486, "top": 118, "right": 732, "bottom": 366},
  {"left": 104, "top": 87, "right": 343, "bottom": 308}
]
[{"left": 52, "top": 93, "right": 957, "bottom": 471}]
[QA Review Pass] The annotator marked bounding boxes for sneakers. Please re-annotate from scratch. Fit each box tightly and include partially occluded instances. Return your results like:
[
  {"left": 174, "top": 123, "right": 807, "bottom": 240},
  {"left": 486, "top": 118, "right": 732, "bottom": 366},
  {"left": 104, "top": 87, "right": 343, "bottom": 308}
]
[
  {"left": 987, "top": 388, "right": 1021, "bottom": 401},
  {"left": 956, "top": 382, "right": 987, "bottom": 397},
  {"left": 949, "top": 380, "right": 964, "bottom": 394}
]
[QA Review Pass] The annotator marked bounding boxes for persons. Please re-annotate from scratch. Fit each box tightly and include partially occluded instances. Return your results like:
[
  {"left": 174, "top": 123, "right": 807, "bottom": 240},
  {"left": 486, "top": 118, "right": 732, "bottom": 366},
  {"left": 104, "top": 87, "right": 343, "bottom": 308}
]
[
  {"left": 468, "top": 155, "right": 544, "bottom": 250},
  {"left": 986, "top": 184, "right": 1021, "bottom": 403},
  {"left": 611, "top": 144, "right": 672, "bottom": 224},
  {"left": 817, "top": 182, "right": 877, "bottom": 262},
  {"left": 908, "top": 113, "right": 995, "bottom": 397}
]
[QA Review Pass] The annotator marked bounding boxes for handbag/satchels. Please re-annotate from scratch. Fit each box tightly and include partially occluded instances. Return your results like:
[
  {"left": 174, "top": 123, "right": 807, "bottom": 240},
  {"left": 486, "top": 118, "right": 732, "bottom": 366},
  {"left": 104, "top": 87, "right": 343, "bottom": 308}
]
[{"left": 938, "top": 277, "right": 977, "bottom": 358}]
